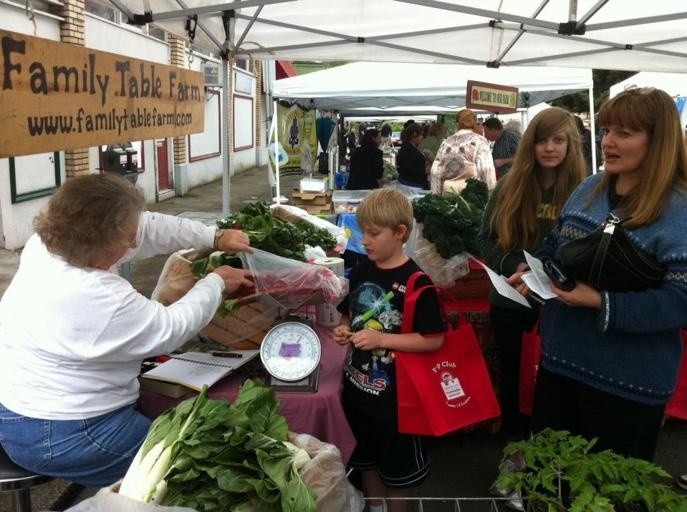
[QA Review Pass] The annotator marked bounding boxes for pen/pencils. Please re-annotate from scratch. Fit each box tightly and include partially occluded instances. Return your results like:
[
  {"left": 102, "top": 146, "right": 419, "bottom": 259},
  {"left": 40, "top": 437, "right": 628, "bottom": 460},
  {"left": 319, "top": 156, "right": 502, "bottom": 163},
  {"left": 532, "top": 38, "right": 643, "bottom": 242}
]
[
  {"left": 212, "top": 352, "right": 243, "bottom": 357},
  {"left": 501, "top": 274, "right": 546, "bottom": 306}
]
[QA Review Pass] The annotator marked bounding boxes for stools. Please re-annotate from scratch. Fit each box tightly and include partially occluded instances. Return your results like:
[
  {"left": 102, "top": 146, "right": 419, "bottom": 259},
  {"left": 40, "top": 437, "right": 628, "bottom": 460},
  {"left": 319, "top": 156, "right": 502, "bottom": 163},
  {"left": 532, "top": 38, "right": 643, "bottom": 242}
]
[{"left": 0, "top": 442, "right": 59, "bottom": 512}]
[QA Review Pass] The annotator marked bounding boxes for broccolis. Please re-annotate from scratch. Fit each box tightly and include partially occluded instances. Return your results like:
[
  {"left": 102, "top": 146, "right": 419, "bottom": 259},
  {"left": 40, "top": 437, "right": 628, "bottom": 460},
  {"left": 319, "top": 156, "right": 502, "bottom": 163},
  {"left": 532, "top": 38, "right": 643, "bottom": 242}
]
[{"left": 412, "top": 178, "right": 488, "bottom": 260}]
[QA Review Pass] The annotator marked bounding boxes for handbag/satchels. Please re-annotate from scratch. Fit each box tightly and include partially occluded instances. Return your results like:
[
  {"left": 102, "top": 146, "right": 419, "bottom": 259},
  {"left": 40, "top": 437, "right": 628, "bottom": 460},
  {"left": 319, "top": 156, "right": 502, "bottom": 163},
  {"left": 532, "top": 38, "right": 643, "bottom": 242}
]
[
  {"left": 518, "top": 318, "right": 541, "bottom": 416},
  {"left": 396, "top": 271, "right": 502, "bottom": 437},
  {"left": 557, "top": 215, "right": 663, "bottom": 292}
]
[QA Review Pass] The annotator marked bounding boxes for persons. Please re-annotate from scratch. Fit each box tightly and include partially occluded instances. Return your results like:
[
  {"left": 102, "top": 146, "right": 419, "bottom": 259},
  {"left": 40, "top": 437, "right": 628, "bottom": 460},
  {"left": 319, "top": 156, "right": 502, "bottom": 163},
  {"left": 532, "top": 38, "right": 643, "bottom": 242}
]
[
  {"left": 337, "top": 114, "right": 603, "bottom": 203},
  {"left": 509, "top": 84, "right": 686, "bottom": 512},
  {"left": 330, "top": 186, "right": 447, "bottom": 511},
  {"left": 1, "top": 171, "right": 267, "bottom": 488},
  {"left": 476, "top": 105, "right": 590, "bottom": 511}
]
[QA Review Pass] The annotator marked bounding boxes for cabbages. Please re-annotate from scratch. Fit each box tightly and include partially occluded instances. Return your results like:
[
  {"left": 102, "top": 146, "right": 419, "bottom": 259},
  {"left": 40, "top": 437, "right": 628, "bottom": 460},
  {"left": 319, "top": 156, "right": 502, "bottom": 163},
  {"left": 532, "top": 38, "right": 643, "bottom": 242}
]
[{"left": 119, "top": 377, "right": 318, "bottom": 512}]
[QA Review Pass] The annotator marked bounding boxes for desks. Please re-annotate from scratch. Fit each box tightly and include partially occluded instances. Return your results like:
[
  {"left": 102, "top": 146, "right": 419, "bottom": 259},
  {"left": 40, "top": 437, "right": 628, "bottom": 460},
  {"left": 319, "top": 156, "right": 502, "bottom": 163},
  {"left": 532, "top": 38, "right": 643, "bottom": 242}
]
[
  {"left": 136, "top": 300, "right": 354, "bottom": 467},
  {"left": 338, "top": 213, "right": 369, "bottom": 256}
]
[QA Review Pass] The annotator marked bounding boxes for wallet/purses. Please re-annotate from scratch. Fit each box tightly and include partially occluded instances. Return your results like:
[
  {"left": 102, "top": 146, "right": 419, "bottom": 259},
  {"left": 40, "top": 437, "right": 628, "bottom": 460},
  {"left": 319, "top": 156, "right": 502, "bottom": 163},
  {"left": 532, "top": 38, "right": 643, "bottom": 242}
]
[{"left": 542, "top": 256, "right": 574, "bottom": 291}]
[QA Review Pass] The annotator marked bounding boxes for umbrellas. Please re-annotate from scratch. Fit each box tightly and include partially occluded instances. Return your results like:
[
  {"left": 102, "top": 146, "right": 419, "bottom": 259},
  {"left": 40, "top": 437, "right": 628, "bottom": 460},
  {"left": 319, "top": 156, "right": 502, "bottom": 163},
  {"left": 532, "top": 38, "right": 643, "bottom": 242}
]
[{"left": 314, "top": 111, "right": 336, "bottom": 157}]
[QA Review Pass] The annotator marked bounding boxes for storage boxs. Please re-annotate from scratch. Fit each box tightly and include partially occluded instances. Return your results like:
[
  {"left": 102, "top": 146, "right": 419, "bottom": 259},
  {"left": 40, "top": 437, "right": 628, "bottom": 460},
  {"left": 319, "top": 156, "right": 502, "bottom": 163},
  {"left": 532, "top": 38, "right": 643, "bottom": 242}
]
[{"left": 291, "top": 178, "right": 371, "bottom": 215}]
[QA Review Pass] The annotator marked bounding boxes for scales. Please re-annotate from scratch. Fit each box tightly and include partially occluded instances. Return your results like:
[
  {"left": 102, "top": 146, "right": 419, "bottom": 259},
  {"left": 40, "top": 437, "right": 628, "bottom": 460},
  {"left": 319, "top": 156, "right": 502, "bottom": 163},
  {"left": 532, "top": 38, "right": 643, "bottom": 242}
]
[{"left": 258, "top": 292, "right": 323, "bottom": 393}]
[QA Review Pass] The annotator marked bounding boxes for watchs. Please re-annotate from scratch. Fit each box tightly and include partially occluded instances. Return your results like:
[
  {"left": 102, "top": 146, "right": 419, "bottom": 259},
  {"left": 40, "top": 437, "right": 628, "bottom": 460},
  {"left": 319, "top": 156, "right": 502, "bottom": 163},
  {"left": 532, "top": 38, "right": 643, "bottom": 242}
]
[{"left": 214, "top": 228, "right": 224, "bottom": 249}]
